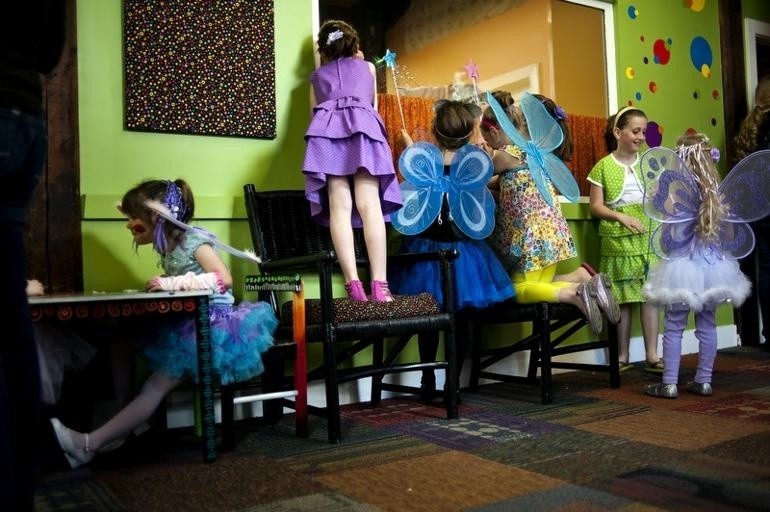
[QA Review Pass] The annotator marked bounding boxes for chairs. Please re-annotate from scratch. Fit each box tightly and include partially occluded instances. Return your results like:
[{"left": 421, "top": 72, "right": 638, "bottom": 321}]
[
  {"left": 181, "top": 272, "right": 311, "bottom": 448},
  {"left": 447, "top": 198, "right": 622, "bottom": 407},
  {"left": 242, "top": 178, "right": 461, "bottom": 442}
]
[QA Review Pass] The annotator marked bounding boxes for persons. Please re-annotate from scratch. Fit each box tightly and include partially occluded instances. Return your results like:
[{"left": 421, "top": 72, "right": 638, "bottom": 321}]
[
  {"left": 477, "top": 90, "right": 623, "bottom": 336},
  {"left": 49, "top": 177, "right": 279, "bottom": 470},
  {"left": 640, "top": 128, "right": 769, "bottom": 400},
  {"left": 400, "top": 99, "right": 518, "bottom": 407},
  {"left": 299, "top": 17, "right": 406, "bottom": 303},
  {"left": 586, "top": 103, "right": 665, "bottom": 374},
  {"left": 735, "top": 77, "right": 770, "bottom": 354},
  {"left": 520, "top": 94, "right": 597, "bottom": 284}
]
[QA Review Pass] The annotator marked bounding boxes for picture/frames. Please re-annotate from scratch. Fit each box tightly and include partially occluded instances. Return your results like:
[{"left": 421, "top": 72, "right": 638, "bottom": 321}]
[{"left": 740, "top": 15, "right": 769, "bottom": 121}]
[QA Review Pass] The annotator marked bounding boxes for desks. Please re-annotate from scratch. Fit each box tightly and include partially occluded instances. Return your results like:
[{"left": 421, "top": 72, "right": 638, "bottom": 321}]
[{"left": 24, "top": 287, "right": 222, "bottom": 468}]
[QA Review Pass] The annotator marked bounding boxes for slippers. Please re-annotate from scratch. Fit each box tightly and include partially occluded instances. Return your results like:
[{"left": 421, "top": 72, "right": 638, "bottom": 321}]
[
  {"left": 618, "top": 361, "right": 635, "bottom": 373},
  {"left": 643, "top": 361, "right": 665, "bottom": 373}
]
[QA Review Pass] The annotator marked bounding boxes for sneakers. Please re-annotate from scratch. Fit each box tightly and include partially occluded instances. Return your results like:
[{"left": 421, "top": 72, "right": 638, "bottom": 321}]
[
  {"left": 443, "top": 380, "right": 464, "bottom": 407},
  {"left": 413, "top": 374, "right": 437, "bottom": 402}
]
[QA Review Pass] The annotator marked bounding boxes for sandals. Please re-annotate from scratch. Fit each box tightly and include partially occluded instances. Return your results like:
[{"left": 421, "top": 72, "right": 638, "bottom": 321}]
[
  {"left": 369, "top": 278, "right": 396, "bottom": 303},
  {"left": 343, "top": 280, "right": 370, "bottom": 303}
]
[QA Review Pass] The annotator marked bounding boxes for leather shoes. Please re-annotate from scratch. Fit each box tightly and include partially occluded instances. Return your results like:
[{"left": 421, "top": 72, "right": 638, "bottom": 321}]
[
  {"left": 644, "top": 382, "right": 679, "bottom": 400},
  {"left": 97, "top": 421, "right": 151, "bottom": 454},
  {"left": 576, "top": 271, "right": 622, "bottom": 337},
  {"left": 678, "top": 381, "right": 713, "bottom": 397},
  {"left": 49, "top": 416, "right": 97, "bottom": 471}
]
